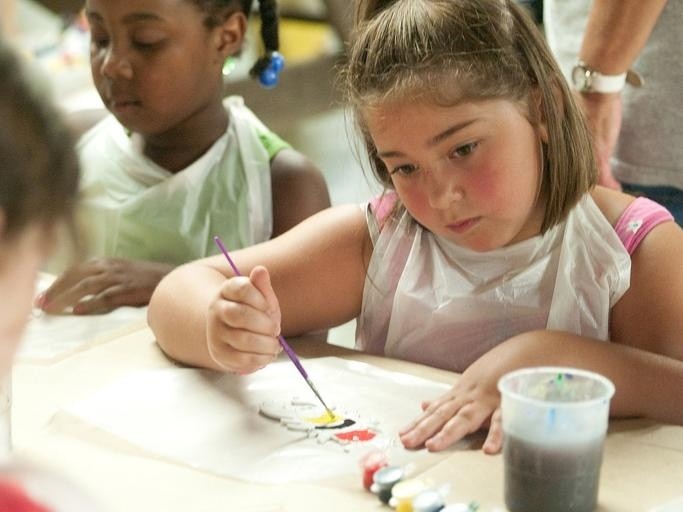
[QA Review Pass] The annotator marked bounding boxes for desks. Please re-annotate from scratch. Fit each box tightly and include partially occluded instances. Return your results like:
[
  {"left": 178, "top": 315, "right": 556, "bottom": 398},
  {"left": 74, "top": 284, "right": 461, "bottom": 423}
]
[{"left": 0, "top": 269, "right": 683, "bottom": 512}]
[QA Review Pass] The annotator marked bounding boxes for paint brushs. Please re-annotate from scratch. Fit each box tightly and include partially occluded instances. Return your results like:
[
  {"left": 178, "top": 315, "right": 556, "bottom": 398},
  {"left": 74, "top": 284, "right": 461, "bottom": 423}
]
[{"left": 215, "top": 236, "right": 335, "bottom": 420}]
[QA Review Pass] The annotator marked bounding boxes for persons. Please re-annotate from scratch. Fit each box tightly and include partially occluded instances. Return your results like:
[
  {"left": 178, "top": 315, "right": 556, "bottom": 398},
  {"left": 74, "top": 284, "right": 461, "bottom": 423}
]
[
  {"left": 2, "top": 40, "right": 79, "bottom": 512},
  {"left": 145, "top": 1, "right": 681, "bottom": 455},
  {"left": 30, "top": 0, "right": 334, "bottom": 344},
  {"left": 542, "top": 0, "right": 682, "bottom": 230}
]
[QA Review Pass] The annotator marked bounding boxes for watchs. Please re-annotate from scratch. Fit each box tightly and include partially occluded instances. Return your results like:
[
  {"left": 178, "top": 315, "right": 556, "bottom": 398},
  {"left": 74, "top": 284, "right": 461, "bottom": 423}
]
[{"left": 567, "top": 62, "right": 646, "bottom": 98}]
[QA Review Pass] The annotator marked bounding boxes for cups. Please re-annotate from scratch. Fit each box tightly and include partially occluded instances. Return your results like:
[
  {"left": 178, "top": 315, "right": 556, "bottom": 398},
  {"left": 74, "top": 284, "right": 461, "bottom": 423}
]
[{"left": 497, "top": 366, "right": 617, "bottom": 507}]
